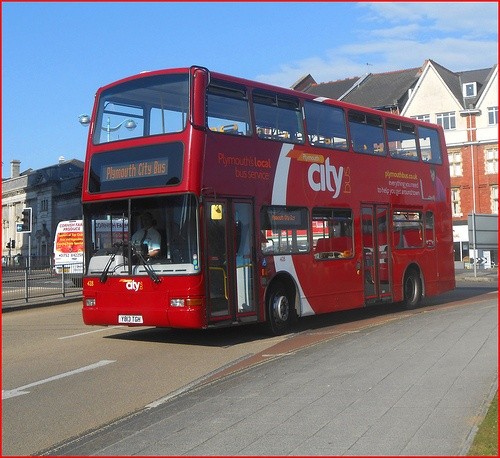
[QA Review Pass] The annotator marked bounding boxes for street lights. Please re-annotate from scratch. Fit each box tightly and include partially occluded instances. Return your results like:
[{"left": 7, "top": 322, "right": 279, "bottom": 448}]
[{"left": 80, "top": 114, "right": 138, "bottom": 140}]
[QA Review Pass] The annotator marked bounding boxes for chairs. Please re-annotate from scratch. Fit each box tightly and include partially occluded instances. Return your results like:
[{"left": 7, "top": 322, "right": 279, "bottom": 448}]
[
  {"left": 209, "top": 123, "right": 416, "bottom": 161},
  {"left": 316, "top": 229, "right": 435, "bottom": 283}
]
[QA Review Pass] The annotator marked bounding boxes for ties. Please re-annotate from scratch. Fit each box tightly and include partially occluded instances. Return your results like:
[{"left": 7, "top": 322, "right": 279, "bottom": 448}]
[{"left": 140, "top": 230, "right": 147, "bottom": 244}]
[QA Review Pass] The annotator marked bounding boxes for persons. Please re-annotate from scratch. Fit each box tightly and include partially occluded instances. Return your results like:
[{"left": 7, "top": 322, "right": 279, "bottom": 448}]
[{"left": 131, "top": 212, "right": 163, "bottom": 258}]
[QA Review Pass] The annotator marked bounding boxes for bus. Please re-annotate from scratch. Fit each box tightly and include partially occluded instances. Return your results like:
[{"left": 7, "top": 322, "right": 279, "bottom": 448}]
[{"left": 79, "top": 64, "right": 455, "bottom": 334}]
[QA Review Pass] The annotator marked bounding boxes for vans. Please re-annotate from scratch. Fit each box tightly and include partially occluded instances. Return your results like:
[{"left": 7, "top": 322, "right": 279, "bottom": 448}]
[{"left": 50, "top": 221, "right": 140, "bottom": 285}]
